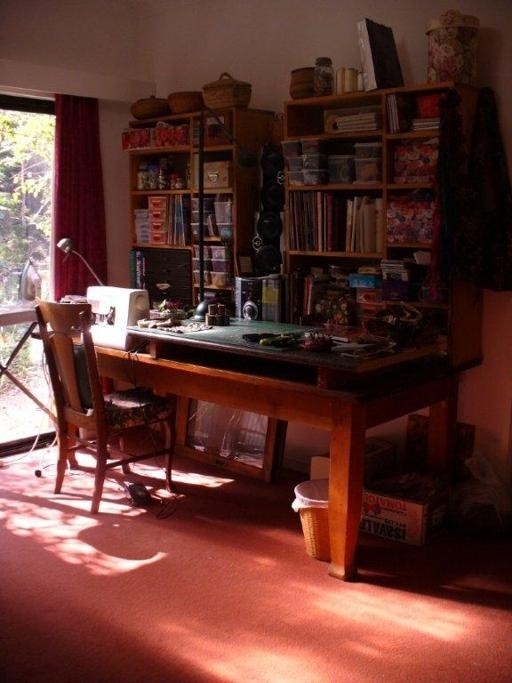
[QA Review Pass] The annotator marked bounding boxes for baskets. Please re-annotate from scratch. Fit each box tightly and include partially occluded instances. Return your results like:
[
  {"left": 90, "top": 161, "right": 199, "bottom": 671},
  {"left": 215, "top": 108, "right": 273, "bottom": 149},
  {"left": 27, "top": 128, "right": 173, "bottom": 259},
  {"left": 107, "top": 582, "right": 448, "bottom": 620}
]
[
  {"left": 201, "top": 71, "right": 251, "bottom": 110},
  {"left": 167, "top": 91, "right": 204, "bottom": 114}
]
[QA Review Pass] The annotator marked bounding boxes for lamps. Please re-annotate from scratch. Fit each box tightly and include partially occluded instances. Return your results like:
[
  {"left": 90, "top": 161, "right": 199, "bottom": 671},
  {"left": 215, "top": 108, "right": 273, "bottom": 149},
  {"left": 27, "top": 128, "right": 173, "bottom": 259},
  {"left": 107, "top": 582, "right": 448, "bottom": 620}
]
[{"left": 57, "top": 236, "right": 104, "bottom": 287}]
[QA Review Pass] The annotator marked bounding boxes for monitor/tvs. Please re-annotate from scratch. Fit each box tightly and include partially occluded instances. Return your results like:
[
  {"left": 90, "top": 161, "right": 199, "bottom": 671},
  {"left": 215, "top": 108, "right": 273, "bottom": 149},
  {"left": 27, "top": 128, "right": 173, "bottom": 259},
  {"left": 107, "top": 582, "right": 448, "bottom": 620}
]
[{"left": 132, "top": 246, "right": 192, "bottom": 312}]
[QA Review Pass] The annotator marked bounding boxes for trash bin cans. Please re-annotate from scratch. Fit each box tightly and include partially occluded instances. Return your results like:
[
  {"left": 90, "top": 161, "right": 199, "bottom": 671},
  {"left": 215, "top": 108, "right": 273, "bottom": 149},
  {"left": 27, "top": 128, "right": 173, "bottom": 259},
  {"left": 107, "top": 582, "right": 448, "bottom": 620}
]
[{"left": 293, "top": 480, "right": 331, "bottom": 560}]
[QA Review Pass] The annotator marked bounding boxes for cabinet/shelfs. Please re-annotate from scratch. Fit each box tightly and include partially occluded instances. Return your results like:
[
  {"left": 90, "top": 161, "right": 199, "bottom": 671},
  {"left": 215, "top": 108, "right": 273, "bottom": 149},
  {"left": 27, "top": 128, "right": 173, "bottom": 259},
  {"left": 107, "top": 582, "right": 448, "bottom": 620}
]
[
  {"left": 124, "top": 108, "right": 272, "bottom": 443},
  {"left": 282, "top": 81, "right": 462, "bottom": 487}
]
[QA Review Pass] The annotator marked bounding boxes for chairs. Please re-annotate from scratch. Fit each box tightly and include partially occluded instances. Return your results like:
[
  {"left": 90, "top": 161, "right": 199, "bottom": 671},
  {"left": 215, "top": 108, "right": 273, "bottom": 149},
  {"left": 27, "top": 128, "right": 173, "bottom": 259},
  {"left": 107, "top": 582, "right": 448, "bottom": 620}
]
[{"left": 33, "top": 302, "right": 178, "bottom": 515}]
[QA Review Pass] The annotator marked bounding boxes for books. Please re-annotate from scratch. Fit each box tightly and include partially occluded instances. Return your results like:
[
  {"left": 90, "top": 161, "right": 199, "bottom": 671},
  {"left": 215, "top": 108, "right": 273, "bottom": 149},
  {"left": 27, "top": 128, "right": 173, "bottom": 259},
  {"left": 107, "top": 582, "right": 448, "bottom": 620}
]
[
  {"left": 331, "top": 98, "right": 439, "bottom": 134},
  {"left": 236, "top": 272, "right": 282, "bottom": 326},
  {"left": 284, "top": 188, "right": 408, "bottom": 320}
]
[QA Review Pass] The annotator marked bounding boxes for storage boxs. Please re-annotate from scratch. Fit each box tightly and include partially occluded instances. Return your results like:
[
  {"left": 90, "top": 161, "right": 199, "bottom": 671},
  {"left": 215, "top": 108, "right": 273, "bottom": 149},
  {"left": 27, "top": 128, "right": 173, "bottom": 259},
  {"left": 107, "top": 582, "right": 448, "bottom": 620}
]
[
  {"left": 360, "top": 473, "right": 463, "bottom": 543},
  {"left": 188, "top": 194, "right": 232, "bottom": 286},
  {"left": 281, "top": 134, "right": 383, "bottom": 188},
  {"left": 132, "top": 194, "right": 167, "bottom": 245},
  {"left": 202, "top": 159, "right": 232, "bottom": 188}
]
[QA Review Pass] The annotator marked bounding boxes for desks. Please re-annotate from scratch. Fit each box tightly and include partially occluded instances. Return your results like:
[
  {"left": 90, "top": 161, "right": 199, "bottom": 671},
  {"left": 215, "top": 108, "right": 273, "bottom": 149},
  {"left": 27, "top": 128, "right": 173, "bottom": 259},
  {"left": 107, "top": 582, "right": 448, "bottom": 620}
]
[{"left": 29, "top": 313, "right": 484, "bottom": 584}]
